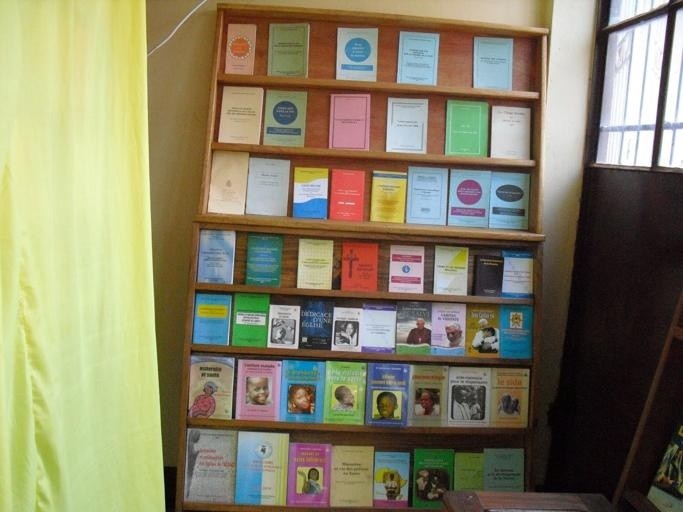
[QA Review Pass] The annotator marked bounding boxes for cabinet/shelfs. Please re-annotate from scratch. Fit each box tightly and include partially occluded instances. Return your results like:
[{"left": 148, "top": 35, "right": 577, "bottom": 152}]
[
  {"left": 169, "top": 3, "right": 552, "bottom": 511},
  {"left": 611, "top": 294, "right": 682, "bottom": 511}
]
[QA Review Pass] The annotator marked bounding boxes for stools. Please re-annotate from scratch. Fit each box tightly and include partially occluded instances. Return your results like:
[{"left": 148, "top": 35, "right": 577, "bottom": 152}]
[{"left": 440, "top": 491, "right": 614, "bottom": 511}]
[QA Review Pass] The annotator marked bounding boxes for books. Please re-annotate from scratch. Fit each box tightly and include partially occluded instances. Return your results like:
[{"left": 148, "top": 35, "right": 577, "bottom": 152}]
[
  {"left": 197, "top": 229, "right": 534, "bottom": 298},
  {"left": 267, "top": 23, "right": 310, "bottom": 80},
  {"left": 225, "top": 24, "right": 256, "bottom": 76},
  {"left": 192, "top": 292, "right": 533, "bottom": 359},
  {"left": 445, "top": 99, "right": 490, "bottom": 157},
  {"left": 491, "top": 105, "right": 530, "bottom": 161},
  {"left": 206, "top": 150, "right": 529, "bottom": 231},
  {"left": 184, "top": 427, "right": 526, "bottom": 511},
  {"left": 473, "top": 36, "right": 513, "bottom": 90},
  {"left": 397, "top": 31, "right": 440, "bottom": 86},
  {"left": 337, "top": 27, "right": 378, "bottom": 82},
  {"left": 187, "top": 356, "right": 529, "bottom": 427},
  {"left": 646, "top": 420, "right": 682, "bottom": 511},
  {"left": 263, "top": 90, "right": 307, "bottom": 147},
  {"left": 386, "top": 97, "right": 428, "bottom": 154},
  {"left": 218, "top": 86, "right": 264, "bottom": 146},
  {"left": 329, "top": 94, "right": 370, "bottom": 150}
]
